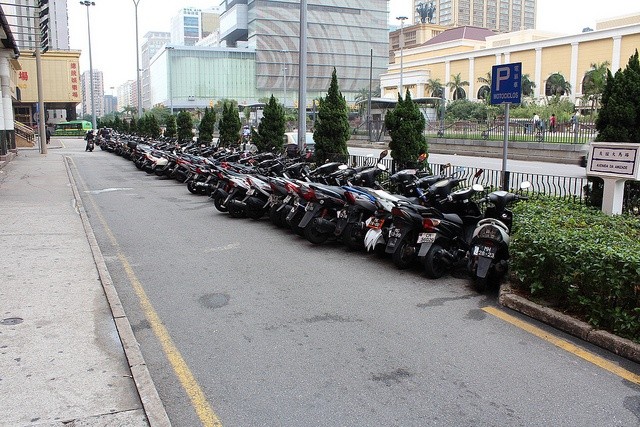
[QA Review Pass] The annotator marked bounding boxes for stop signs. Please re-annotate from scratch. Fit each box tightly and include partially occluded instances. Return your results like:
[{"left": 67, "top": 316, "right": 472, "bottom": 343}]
[{"left": 490, "top": 62, "right": 520, "bottom": 104}]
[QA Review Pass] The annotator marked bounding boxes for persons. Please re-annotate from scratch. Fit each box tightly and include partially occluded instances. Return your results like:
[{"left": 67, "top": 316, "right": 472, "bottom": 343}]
[
  {"left": 571, "top": 111, "right": 579, "bottom": 133},
  {"left": 549, "top": 113, "right": 556, "bottom": 132},
  {"left": 533, "top": 112, "right": 540, "bottom": 132},
  {"left": 85, "top": 130, "right": 94, "bottom": 151},
  {"left": 101, "top": 125, "right": 109, "bottom": 137},
  {"left": 46, "top": 130, "right": 51, "bottom": 143}
]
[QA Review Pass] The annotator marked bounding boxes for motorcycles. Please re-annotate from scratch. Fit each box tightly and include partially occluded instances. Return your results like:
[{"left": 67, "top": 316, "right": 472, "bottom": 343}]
[
  {"left": 464, "top": 181, "right": 534, "bottom": 292},
  {"left": 96, "top": 130, "right": 156, "bottom": 160},
  {"left": 333, "top": 151, "right": 433, "bottom": 252},
  {"left": 130, "top": 135, "right": 220, "bottom": 182},
  {"left": 365, "top": 163, "right": 449, "bottom": 260},
  {"left": 385, "top": 177, "right": 466, "bottom": 270},
  {"left": 85, "top": 136, "right": 94, "bottom": 152},
  {"left": 297, "top": 149, "right": 398, "bottom": 246},
  {"left": 189, "top": 146, "right": 313, "bottom": 237},
  {"left": 416, "top": 185, "right": 490, "bottom": 280}
]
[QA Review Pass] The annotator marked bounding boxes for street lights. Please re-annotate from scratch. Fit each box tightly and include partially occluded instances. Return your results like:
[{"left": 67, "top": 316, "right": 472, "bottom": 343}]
[{"left": 79, "top": 0, "right": 96, "bottom": 135}]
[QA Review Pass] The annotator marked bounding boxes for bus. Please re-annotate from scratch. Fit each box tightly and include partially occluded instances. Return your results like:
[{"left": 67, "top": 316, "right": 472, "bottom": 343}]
[{"left": 46, "top": 119, "right": 92, "bottom": 136}]
